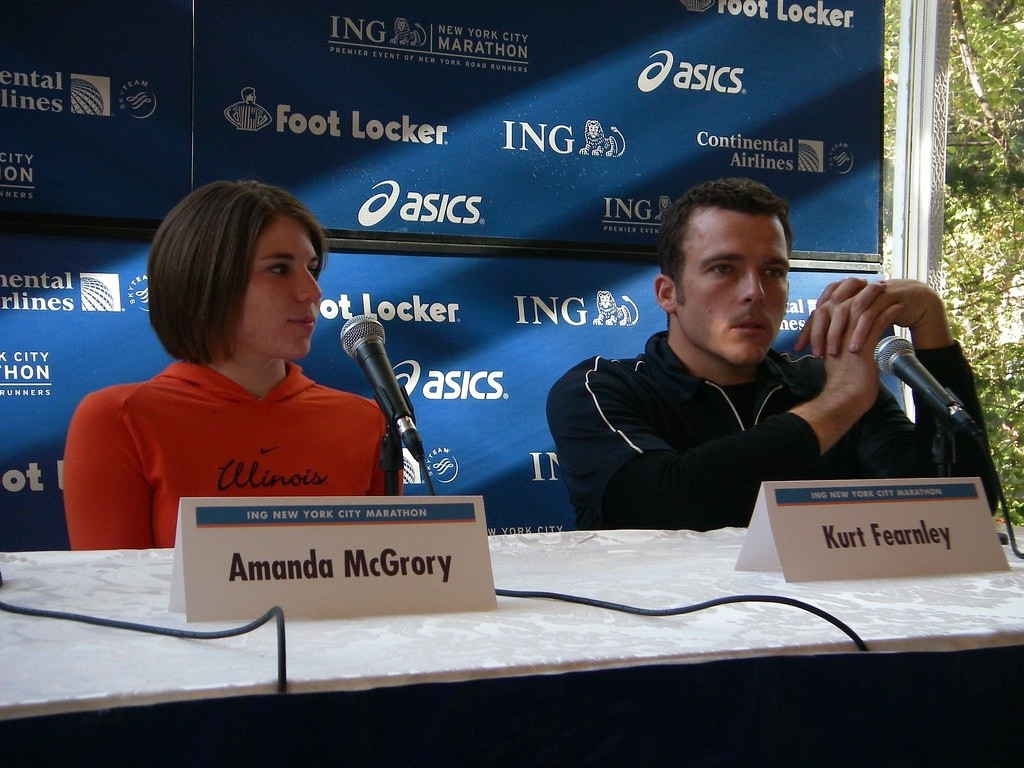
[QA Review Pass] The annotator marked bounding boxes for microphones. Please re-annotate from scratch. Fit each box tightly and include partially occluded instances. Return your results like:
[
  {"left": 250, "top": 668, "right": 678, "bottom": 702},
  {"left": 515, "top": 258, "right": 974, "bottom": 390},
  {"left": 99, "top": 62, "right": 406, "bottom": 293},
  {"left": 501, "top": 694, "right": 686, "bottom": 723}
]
[
  {"left": 873, "top": 336, "right": 983, "bottom": 439},
  {"left": 340, "top": 315, "right": 426, "bottom": 463}
]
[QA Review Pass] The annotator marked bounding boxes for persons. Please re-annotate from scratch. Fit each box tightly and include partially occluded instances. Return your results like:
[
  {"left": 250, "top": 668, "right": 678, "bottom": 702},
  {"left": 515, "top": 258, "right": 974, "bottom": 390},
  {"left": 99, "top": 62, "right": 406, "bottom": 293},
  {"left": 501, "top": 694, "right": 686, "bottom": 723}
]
[
  {"left": 63, "top": 178, "right": 404, "bottom": 551},
  {"left": 544, "top": 176, "right": 998, "bottom": 531}
]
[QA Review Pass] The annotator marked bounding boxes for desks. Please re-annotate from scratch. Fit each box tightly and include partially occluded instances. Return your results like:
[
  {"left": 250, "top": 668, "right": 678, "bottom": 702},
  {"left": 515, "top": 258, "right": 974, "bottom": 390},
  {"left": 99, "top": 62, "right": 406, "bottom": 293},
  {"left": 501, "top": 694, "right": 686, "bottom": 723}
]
[{"left": 0, "top": 528, "right": 1024, "bottom": 768}]
